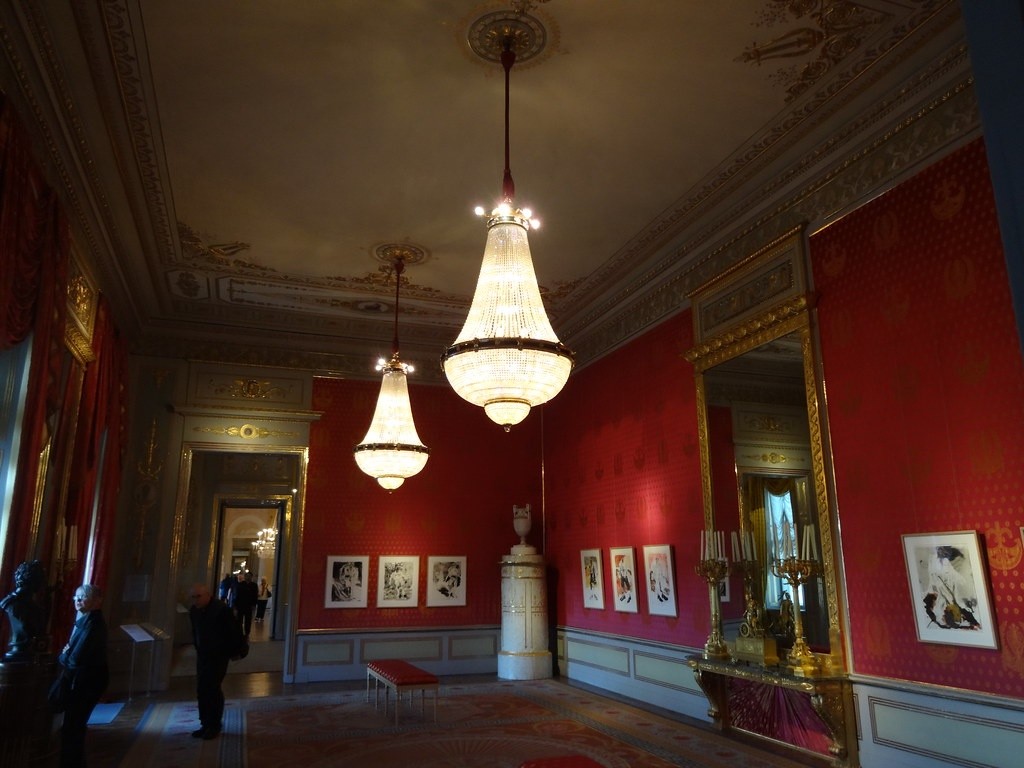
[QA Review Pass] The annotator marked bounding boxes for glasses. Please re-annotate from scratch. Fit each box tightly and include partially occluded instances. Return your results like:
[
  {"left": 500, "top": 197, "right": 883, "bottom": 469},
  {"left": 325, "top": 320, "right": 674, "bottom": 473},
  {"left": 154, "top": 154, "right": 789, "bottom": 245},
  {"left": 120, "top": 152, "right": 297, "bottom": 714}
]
[
  {"left": 190, "top": 592, "right": 207, "bottom": 601},
  {"left": 73, "top": 594, "right": 89, "bottom": 601}
]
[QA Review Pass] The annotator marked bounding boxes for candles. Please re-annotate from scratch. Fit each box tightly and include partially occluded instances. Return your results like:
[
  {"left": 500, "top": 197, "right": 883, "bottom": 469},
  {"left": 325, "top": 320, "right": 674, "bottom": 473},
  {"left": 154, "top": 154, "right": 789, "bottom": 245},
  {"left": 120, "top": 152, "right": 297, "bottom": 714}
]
[
  {"left": 802, "top": 523, "right": 818, "bottom": 561},
  {"left": 773, "top": 521, "right": 800, "bottom": 561},
  {"left": 701, "top": 529, "right": 727, "bottom": 562},
  {"left": 731, "top": 530, "right": 758, "bottom": 562}
]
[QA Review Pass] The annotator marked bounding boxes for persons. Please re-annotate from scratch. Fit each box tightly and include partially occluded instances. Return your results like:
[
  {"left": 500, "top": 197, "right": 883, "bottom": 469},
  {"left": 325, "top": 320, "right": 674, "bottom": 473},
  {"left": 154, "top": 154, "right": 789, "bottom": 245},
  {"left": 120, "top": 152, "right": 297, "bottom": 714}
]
[
  {"left": 219, "top": 572, "right": 272, "bottom": 640},
  {"left": 0, "top": 560, "right": 51, "bottom": 659},
  {"left": 58, "top": 584, "right": 109, "bottom": 768},
  {"left": 189, "top": 583, "right": 249, "bottom": 739}
]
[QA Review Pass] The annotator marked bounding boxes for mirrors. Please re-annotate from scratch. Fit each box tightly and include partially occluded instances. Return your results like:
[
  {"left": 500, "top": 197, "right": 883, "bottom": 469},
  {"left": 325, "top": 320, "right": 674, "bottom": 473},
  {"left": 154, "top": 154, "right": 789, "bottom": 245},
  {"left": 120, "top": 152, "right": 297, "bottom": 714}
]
[{"left": 693, "top": 309, "right": 845, "bottom": 676}]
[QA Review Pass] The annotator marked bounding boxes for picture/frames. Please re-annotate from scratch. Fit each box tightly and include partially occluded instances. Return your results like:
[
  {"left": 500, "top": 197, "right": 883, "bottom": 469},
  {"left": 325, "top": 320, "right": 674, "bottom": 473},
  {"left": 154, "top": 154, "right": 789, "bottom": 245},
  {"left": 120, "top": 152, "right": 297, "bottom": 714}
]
[
  {"left": 609, "top": 546, "right": 638, "bottom": 614},
  {"left": 580, "top": 548, "right": 605, "bottom": 610},
  {"left": 376, "top": 555, "right": 420, "bottom": 608},
  {"left": 324, "top": 555, "right": 368, "bottom": 608},
  {"left": 900, "top": 530, "right": 998, "bottom": 650},
  {"left": 642, "top": 544, "right": 678, "bottom": 618},
  {"left": 427, "top": 555, "right": 466, "bottom": 607}
]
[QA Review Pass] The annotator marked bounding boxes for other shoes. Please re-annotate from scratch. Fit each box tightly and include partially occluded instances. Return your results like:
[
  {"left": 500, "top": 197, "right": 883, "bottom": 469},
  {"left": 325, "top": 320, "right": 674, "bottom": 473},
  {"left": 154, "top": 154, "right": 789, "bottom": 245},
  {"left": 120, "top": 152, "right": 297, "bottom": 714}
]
[
  {"left": 192, "top": 723, "right": 222, "bottom": 741},
  {"left": 254, "top": 617, "right": 259, "bottom": 623},
  {"left": 261, "top": 619, "right": 264, "bottom": 622}
]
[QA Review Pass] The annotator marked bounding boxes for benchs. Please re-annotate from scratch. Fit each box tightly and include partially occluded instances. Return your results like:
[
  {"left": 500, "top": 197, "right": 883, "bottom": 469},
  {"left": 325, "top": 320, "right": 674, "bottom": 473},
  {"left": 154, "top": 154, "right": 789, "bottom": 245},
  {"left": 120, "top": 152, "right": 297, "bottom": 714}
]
[{"left": 366, "top": 659, "right": 440, "bottom": 725}]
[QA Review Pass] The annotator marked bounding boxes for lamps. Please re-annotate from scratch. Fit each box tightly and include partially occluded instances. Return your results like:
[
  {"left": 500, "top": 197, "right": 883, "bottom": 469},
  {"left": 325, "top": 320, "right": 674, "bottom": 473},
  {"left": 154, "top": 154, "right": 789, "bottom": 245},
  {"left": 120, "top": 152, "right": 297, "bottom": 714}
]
[
  {"left": 352, "top": 258, "right": 431, "bottom": 494},
  {"left": 440, "top": 34, "right": 577, "bottom": 433},
  {"left": 251, "top": 529, "right": 276, "bottom": 553}
]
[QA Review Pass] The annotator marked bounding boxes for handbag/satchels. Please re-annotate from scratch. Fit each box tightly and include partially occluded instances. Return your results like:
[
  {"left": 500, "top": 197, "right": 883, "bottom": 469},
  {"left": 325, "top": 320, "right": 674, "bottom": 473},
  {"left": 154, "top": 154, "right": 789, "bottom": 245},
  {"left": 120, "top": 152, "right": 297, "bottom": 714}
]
[
  {"left": 48, "top": 667, "right": 71, "bottom": 713},
  {"left": 267, "top": 584, "right": 272, "bottom": 598}
]
